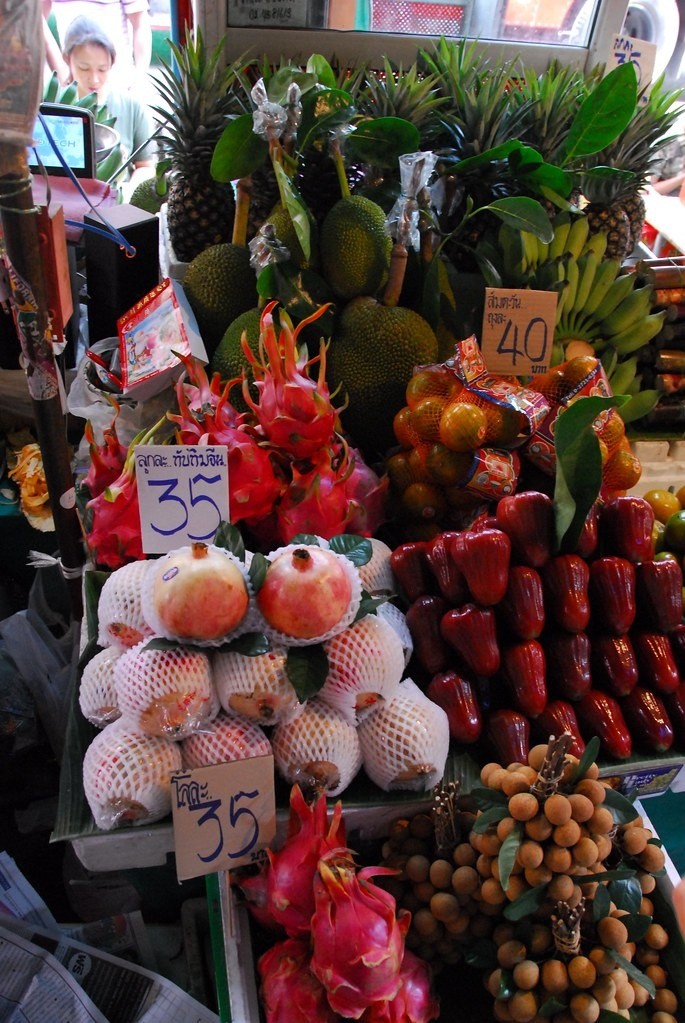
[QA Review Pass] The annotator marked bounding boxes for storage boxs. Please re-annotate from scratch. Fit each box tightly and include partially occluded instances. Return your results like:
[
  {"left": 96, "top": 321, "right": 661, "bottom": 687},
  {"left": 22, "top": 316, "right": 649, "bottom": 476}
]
[{"left": 209, "top": 789, "right": 685, "bottom": 1023}]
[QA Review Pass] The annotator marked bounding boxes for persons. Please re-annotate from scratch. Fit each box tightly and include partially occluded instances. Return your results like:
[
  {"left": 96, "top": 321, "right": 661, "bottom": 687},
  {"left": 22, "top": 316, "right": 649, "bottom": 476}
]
[
  {"left": 647, "top": 139, "right": 685, "bottom": 194},
  {"left": 41, "top": 0, "right": 152, "bottom": 97},
  {"left": 62, "top": 17, "right": 155, "bottom": 189}
]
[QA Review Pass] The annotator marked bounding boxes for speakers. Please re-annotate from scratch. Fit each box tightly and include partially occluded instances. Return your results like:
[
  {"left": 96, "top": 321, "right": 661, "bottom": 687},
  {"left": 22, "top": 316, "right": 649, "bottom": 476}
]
[{"left": 83, "top": 204, "right": 159, "bottom": 351}]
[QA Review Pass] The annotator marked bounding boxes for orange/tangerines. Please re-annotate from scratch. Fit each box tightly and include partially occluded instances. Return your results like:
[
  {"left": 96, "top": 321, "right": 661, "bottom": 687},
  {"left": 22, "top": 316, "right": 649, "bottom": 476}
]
[{"left": 386, "top": 356, "right": 685, "bottom": 606}]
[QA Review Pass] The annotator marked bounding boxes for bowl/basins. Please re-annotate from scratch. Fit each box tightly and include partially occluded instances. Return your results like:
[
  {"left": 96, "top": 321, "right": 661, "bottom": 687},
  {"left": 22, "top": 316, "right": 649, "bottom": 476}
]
[{"left": 94, "top": 123, "right": 121, "bottom": 162}]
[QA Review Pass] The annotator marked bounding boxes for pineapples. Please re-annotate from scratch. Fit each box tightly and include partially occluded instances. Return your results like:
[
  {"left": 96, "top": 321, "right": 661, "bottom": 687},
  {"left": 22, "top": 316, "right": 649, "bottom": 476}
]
[{"left": 150, "top": 16, "right": 685, "bottom": 265}]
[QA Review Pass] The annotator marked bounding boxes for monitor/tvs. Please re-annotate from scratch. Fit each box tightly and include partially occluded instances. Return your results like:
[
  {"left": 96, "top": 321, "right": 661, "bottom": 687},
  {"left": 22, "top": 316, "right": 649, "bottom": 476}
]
[{"left": 28, "top": 102, "right": 99, "bottom": 181}]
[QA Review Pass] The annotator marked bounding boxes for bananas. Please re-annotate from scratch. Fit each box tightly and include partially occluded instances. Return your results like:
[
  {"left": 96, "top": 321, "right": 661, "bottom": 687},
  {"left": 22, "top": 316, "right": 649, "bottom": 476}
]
[{"left": 514, "top": 213, "right": 666, "bottom": 424}]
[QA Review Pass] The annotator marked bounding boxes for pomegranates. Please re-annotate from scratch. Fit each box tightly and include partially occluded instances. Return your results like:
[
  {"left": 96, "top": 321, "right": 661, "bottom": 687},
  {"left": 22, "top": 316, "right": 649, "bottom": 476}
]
[{"left": 77, "top": 540, "right": 447, "bottom": 829}]
